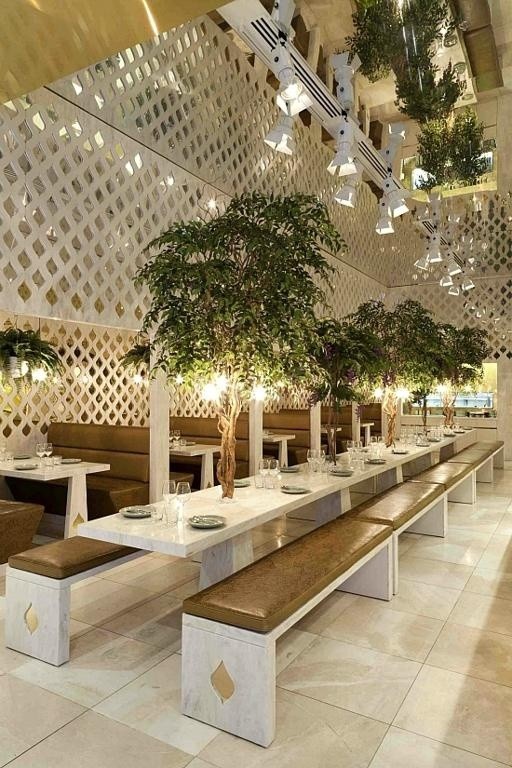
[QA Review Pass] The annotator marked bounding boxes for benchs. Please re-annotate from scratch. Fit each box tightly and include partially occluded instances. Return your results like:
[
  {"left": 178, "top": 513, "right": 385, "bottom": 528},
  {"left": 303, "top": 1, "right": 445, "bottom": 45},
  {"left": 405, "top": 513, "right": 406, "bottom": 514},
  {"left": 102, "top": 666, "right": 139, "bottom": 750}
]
[{"left": 0, "top": 401, "right": 505, "bottom": 749}]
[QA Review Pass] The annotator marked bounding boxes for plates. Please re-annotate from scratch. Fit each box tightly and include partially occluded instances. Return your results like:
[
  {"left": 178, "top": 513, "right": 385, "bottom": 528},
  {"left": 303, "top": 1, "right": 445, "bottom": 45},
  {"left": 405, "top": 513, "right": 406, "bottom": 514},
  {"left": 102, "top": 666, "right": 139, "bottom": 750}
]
[
  {"left": 120, "top": 505, "right": 157, "bottom": 518},
  {"left": 329, "top": 469, "right": 353, "bottom": 476},
  {"left": 279, "top": 466, "right": 300, "bottom": 473},
  {"left": 13, "top": 453, "right": 37, "bottom": 471},
  {"left": 281, "top": 484, "right": 309, "bottom": 494},
  {"left": 188, "top": 515, "right": 226, "bottom": 527},
  {"left": 61, "top": 458, "right": 82, "bottom": 464},
  {"left": 233, "top": 478, "right": 251, "bottom": 488}
]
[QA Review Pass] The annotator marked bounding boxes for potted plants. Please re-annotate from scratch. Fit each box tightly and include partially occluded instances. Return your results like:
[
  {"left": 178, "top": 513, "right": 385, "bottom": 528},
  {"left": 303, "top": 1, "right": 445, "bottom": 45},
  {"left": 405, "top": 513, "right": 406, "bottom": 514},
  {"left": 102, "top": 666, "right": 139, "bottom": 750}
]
[
  {"left": 1, "top": 317, "right": 64, "bottom": 394},
  {"left": 119, "top": 186, "right": 348, "bottom": 503},
  {"left": 306, "top": 300, "right": 492, "bottom": 467},
  {"left": 343, "top": 0, "right": 500, "bottom": 194}
]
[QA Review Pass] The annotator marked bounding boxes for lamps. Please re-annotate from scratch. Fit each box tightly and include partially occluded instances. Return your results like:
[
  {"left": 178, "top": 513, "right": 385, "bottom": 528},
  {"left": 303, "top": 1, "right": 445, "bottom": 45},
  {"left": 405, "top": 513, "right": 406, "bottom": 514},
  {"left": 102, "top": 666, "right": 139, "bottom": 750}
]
[
  {"left": 264, "top": 36, "right": 477, "bottom": 297},
  {"left": 270, "top": 0, "right": 476, "bottom": 264}
]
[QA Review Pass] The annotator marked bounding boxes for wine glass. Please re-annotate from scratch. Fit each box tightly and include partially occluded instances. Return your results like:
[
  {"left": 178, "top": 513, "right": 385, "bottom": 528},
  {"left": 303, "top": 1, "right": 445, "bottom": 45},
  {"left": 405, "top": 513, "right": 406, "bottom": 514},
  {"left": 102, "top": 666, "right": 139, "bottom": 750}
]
[
  {"left": 169, "top": 430, "right": 181, "bottom": 447},
  {"left": 346, "top": 422, "right": 463, "bottom": 465},
  {"left": 160, "top": 480, "right": 192, "bottom": 528},
  {"left": 256, "top": 458, "right": 279, "bottom": 489},
  {"left": 306, "top": 449, "right": 325, "bottom": 474},
  {"left": 35, "top": 442, "right": 53, "bottom": 470}
]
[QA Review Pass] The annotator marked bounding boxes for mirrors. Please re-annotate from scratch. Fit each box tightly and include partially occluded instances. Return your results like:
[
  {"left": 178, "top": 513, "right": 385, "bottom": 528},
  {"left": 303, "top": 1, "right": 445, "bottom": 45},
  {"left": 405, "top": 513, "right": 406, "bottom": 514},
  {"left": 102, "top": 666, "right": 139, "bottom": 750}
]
[{"left": 43, "top": 1, "right": 512, "bottom": 288}]
